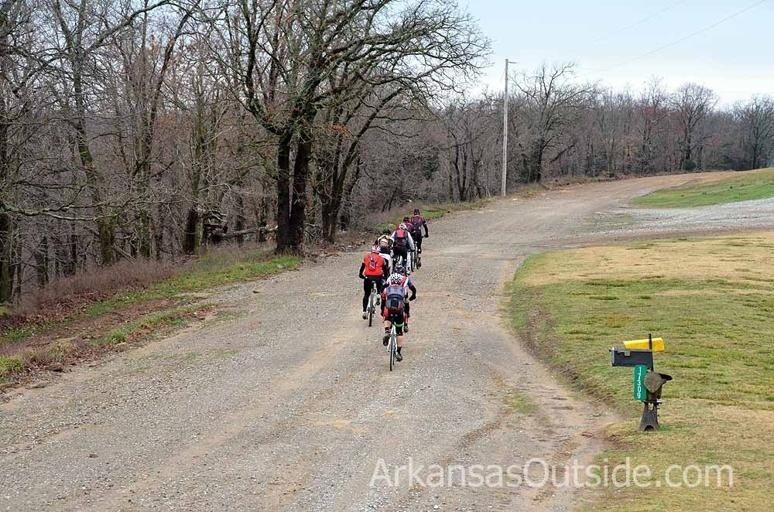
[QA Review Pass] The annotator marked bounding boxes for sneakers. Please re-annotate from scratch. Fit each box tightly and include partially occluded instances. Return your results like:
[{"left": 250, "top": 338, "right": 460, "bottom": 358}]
[
  {"left": 394, "top": 350, "right": 402, "bottom": 361},
  {"left": 383, "top": 333, "right": 389, "bottom": 346},
  {"left": 404, "top": 325, "right": 408, "bottom": 333},
  {"left": 362, "top": 312, "right": 368, "bottom": 320}
]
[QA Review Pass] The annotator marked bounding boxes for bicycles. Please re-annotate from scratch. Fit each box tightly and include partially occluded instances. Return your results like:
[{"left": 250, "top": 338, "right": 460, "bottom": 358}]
[
  {"left": 386, "top": 310, "right": 409, "bottom": 372},
  {"left": 362, "top": 235, "right": 426, "bottom": 327}
]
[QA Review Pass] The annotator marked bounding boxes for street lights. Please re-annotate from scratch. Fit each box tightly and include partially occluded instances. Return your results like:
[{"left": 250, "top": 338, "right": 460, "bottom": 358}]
[{"left": 499, "top": 58, "right": 518, "bottom": 200}]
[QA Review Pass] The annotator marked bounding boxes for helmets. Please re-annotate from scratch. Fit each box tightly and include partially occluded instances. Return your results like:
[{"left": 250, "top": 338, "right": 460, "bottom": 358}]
[{"left": 370, "top": 206, "right": 420, "bottom": 285}]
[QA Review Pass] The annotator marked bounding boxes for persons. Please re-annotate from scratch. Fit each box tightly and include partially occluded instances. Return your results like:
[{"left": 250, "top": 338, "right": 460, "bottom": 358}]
[{"left": 359, "top": 208, "right": 429, "bottom": 362}]
